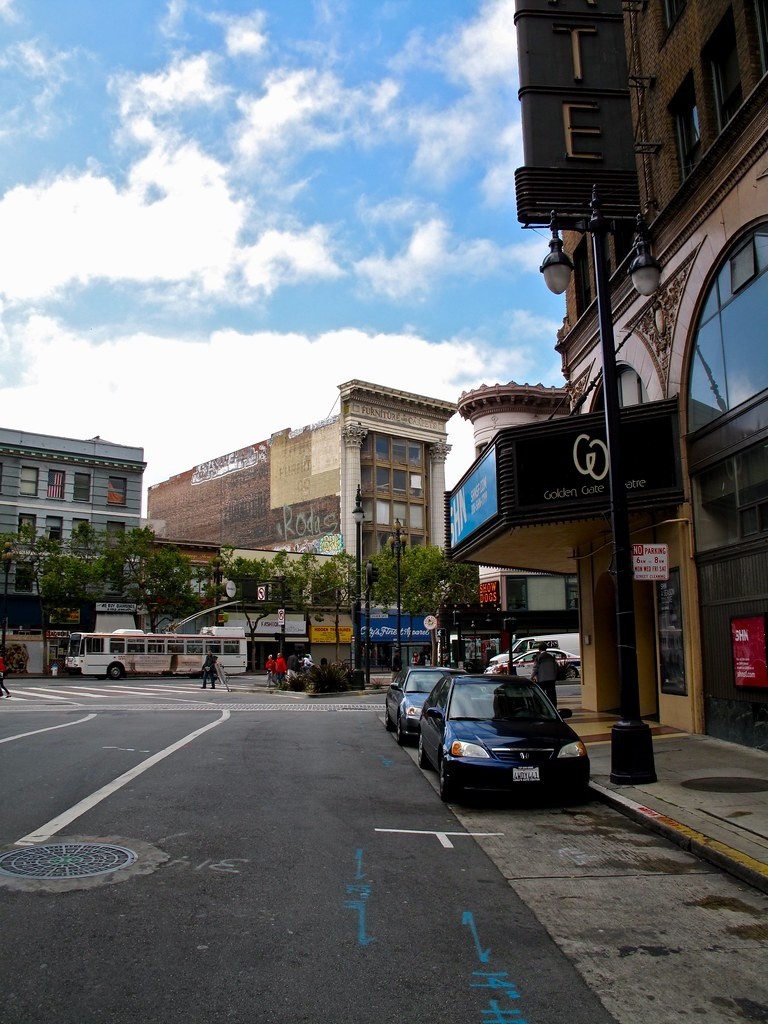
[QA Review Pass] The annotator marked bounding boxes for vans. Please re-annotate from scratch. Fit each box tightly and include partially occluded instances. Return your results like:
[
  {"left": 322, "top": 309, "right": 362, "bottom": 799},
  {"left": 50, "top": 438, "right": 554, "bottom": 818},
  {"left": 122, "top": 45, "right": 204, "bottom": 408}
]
[{"left": 489, "top": 631, "right": 581, "bottom": 665}]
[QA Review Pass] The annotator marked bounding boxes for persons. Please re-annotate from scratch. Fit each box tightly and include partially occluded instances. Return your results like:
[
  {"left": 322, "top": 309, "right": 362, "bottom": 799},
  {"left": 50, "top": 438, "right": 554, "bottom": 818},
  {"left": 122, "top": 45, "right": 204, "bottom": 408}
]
[
  {"left": 202, "top": 651, "right": 217, "bottom": 689},
  {"left": 531, "top": 643, "right": 559, "bottom": 710},
  {"left": 416, "top": 652, "right": 425, "bottom": 665},
  {"left": 287, "top": 651, "right": 301, "bottom": 674},
  {"left": 265, "top": 655, "right": 278, "bottom": 687},
  {"left": 391, "top": 650, "right": 401, "bottom": 682},
  {"left": 275, "top": 653, "right": 286, "bottom": 685},
  {"left": 0, "top": 650, "right": 11, "bottom": 698},
  {"left": 411, "top": 651, "right": 419, "bottom": 666}
]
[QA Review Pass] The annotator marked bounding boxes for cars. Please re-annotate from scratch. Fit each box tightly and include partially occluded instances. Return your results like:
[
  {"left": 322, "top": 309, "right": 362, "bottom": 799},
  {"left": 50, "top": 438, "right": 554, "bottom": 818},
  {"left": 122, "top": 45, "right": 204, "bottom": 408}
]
[
  {"left": 483, "top": 648, "right": 584, "bottom": 680},
  {"left": 416, "top": 673, "right": 592, "bottom": 805},
  {"left": 386, "top": 668, "right": 472, "bottom": 741}
]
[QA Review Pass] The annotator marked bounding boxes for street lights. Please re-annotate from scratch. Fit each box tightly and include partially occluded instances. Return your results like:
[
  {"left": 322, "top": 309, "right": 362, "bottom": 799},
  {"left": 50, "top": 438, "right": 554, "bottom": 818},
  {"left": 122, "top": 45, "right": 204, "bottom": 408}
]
[
  {"left": 1, "top": 538, "right": 15, "bottom": 651},
  {"left": 210, "top": 548, "right": 226, "bottom": 628},
  {"left": 387, "top": 518, "right": 407, "bottom": 666},
  {"left": 352, "top": 484, "right": 365, "bottom": 669},
  {"left": 541, "top": 185, "right": 670, "bottom": 783}
]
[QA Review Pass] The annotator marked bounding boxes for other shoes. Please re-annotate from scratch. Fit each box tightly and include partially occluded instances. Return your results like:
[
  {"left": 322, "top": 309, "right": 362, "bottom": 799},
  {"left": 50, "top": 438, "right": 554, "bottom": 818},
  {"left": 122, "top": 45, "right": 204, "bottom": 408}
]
[
  {"left": 5, "top": 696, "right": 12, "bottom": 698},
  {"left": 201, "top": 687, "right": 206, "bottom": 689},
  {"left": 0, "top": 692, "right": 3, "bottom": 696},
  {"left": 210, "top": 688, "right": 215, "bottom": 689}
]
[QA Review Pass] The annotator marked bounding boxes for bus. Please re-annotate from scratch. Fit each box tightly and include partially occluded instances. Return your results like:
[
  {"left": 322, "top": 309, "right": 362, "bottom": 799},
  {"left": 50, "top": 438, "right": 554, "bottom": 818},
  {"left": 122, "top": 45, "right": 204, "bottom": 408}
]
[{"left": 64, "top": 626, "right": 249, "bottom": 681}]
